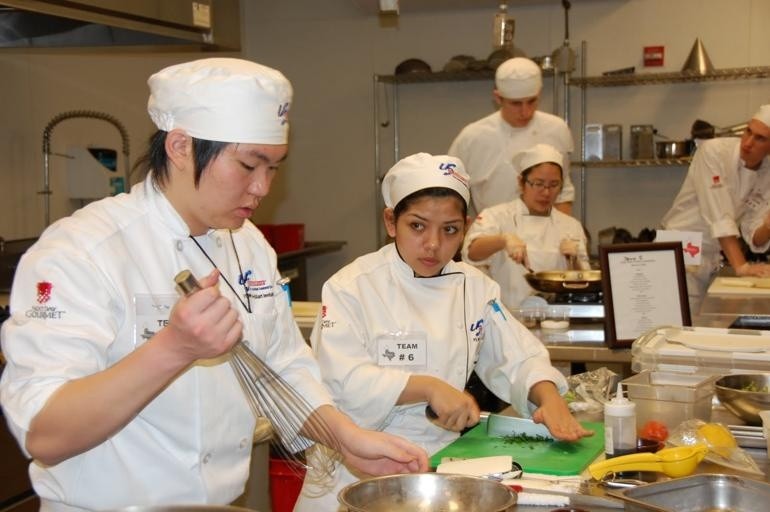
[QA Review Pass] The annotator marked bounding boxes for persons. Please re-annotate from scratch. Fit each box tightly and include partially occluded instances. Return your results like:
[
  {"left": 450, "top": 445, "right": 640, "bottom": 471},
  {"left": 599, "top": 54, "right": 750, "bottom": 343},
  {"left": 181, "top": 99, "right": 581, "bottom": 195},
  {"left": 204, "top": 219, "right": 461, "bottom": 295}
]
[
  {"left": 447, "top": 57, "right": 576, "bottom": 234},
  {"left": 291, "top": 151, "right": 595, "bottom": 512},
  {"left": 0, "top": 57, "right": 429, "bottom": 512},
  {"left": 661, "top": 103, "right": 770, "bottom": 317},
  {"left": 460, "top": 144, "right": 592, "bottom": 311}
]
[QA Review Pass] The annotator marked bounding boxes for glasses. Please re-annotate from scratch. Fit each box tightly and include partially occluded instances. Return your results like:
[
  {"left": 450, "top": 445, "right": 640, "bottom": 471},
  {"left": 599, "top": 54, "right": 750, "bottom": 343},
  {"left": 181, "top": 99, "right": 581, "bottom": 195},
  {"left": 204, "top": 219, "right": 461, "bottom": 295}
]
[{"left": 524, "top": 178, "right": 561, "bottom": 191}]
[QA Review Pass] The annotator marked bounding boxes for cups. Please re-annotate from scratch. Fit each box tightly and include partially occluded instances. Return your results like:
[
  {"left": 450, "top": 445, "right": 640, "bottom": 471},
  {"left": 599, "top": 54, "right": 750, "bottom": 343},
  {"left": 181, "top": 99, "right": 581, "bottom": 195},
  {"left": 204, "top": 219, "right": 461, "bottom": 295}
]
[{"left": 636, "top": 439, "right": 661, "bottom": 482}]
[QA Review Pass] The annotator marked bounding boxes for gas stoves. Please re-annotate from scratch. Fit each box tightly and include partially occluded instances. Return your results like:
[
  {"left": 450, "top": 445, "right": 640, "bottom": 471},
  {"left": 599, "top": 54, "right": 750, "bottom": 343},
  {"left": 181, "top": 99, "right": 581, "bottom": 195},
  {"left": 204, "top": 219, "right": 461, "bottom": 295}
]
[{"left": 513, "top": 292, "right": 605, "bottom": 320}]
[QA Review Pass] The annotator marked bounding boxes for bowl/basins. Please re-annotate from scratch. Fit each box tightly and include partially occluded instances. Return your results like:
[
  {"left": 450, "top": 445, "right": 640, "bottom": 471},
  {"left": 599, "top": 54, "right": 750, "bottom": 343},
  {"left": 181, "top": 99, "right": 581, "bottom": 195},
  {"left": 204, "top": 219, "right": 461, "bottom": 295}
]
[
  {"left": 259, "top": 222, "right": 305, "bottom": 254},
  {"left": 510, "top": 306, "right": 573, "bottom": 330},
  {"left": 654, "top": 140, "right": 690, "bottom": 160},
  {"left": 336, "top": 471, "right": 518, "bottom": 512},
  {"left": 712, "top": 373, "right": 769, "bottom": 424}
]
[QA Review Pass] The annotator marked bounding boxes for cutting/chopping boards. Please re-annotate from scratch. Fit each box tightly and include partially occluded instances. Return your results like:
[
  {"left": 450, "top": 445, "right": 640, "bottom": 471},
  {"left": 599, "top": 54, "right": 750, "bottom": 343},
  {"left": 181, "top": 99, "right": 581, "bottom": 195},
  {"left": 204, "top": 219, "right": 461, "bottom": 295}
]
[
  {"left": 427, "top": 417, "right": 607, "bottom": 475},
  {"left": 708, "top": 275, "right": 770, "bottom": 297}
]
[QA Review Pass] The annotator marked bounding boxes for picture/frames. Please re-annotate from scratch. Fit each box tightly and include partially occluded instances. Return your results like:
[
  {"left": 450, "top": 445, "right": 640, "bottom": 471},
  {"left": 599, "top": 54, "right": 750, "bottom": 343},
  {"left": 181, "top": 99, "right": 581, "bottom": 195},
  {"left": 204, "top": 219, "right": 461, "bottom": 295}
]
[{"left": 596, "top": 240, "right": 693, "bottom": 349}]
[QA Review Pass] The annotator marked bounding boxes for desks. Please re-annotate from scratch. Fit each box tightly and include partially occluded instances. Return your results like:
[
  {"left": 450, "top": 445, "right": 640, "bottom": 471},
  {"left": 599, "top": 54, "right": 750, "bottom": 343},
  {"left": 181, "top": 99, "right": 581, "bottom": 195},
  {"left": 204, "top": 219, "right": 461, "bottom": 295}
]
[
  {"left": 347, "top": 384, "right": 769, "bottom": 512},
  {"left": 701, "top": 266, "right": 770, "bottom": 317}
]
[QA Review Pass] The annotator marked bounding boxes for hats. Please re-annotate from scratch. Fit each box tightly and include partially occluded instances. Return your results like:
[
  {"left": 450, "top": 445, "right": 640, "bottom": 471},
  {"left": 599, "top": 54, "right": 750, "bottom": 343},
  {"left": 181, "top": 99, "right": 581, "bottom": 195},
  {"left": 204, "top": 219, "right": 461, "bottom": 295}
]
[
  {"left": 146, "top": 57, "right": 293, "bottom": 145},
  {"left": 495, "top": 58, "right": 543, "bottom": 99},
  {"left": 752, "top": 105, "right": 770, "bottom": 128},
  {"left": 381, "top": 152, "right": 472, "bottom": 212},
  {"left": 512, "top": 144, "right": 563, "bottom": 175}
]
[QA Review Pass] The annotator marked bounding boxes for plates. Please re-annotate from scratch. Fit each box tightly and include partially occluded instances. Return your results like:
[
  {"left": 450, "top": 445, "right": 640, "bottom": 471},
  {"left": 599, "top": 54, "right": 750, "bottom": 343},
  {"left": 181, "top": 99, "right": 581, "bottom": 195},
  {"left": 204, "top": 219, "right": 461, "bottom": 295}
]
[{"left": 729, "top": 315, "right": 769, "bottom": 330}]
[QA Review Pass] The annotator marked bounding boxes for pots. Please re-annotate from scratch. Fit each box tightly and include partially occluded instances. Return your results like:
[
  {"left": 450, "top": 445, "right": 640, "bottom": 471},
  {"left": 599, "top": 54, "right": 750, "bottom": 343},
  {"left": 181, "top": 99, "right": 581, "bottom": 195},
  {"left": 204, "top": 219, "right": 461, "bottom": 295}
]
[{"left": 522, "top": 251, "right": 603, "bottom": 295}]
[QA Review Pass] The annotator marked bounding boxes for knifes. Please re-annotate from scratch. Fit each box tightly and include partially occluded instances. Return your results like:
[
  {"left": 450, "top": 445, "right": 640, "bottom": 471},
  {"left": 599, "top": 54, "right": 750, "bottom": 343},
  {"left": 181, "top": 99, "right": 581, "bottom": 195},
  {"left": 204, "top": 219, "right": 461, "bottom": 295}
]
[{"left": 424, "top": 403, "right": 556, "bottom": 442}]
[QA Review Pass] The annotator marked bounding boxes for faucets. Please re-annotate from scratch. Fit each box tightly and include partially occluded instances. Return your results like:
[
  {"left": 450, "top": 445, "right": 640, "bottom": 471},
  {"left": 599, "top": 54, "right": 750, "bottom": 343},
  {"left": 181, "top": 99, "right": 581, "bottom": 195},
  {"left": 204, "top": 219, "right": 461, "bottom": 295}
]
[{"left": 36, "top": 110, "right": 133, "bottom": 228}]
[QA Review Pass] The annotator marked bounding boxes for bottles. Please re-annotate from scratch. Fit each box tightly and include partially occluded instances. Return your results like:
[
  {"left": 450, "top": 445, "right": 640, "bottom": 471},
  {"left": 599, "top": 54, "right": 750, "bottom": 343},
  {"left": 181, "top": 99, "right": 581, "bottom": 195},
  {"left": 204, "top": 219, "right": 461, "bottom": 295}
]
[
  {"left": 603, "top": 383, "right": 639, "bottom": 478},
  {"left": 489, "top": 3, "right": 515, "bottom": 48}
]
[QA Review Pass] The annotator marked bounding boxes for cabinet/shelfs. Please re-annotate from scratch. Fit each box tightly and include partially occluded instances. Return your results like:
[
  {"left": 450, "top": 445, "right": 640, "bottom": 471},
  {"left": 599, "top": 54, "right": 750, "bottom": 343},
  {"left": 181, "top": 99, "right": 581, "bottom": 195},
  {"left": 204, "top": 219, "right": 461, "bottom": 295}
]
[
  {"left": 568, "top": 67, "right": 770, "bottom": 168},
  {"left": 372, "top": 62, "right": 568, "bottom": 253}
]
